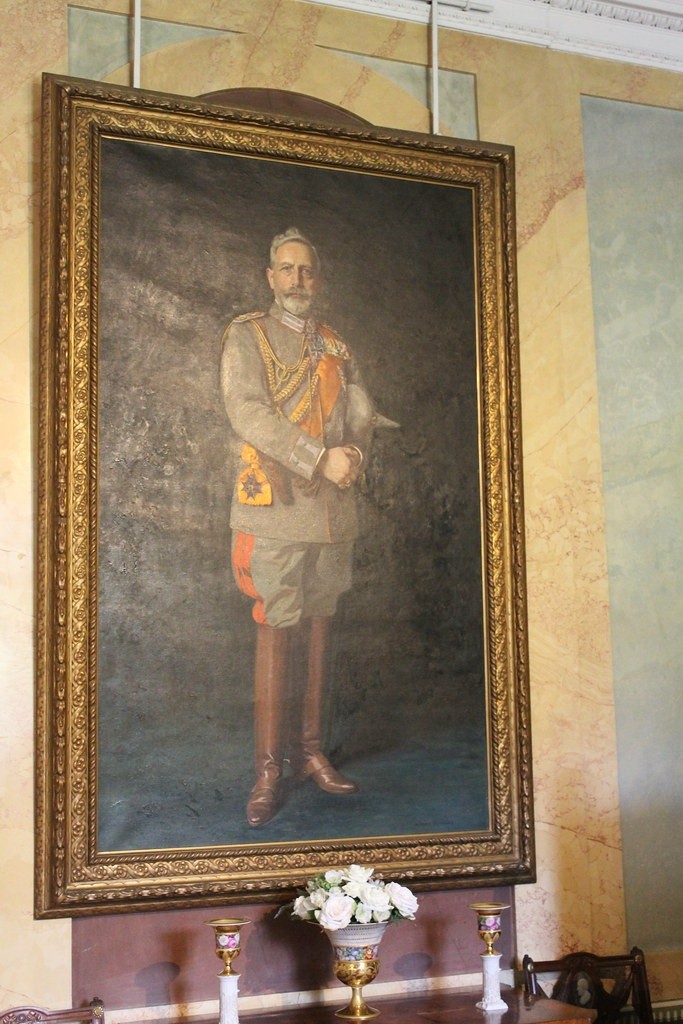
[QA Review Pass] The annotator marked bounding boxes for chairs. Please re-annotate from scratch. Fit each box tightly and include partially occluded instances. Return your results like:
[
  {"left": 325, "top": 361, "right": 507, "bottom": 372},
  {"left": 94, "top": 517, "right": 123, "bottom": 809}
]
[{"left": 521, "top": 946, "right": 656, "bottom": 1024}]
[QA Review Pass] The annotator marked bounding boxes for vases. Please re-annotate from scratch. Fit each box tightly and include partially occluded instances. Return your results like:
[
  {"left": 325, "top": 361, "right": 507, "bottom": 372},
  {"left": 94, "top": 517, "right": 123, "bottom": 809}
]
[{"left": 317, "top": 918, "right": 390, "bottom": 1020}]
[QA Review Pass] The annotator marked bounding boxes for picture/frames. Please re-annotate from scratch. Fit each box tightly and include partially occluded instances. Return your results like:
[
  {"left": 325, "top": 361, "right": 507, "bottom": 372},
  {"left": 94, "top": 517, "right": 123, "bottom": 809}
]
[{"left": 34, "top": 69, "right": 538, "bottom": 923}]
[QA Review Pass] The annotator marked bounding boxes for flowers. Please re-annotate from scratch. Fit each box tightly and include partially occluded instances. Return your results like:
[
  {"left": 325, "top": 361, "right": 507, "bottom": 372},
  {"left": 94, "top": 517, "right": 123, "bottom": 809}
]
[{"left": 271, "top": 863, "right": 420, "bottom": 931}]
